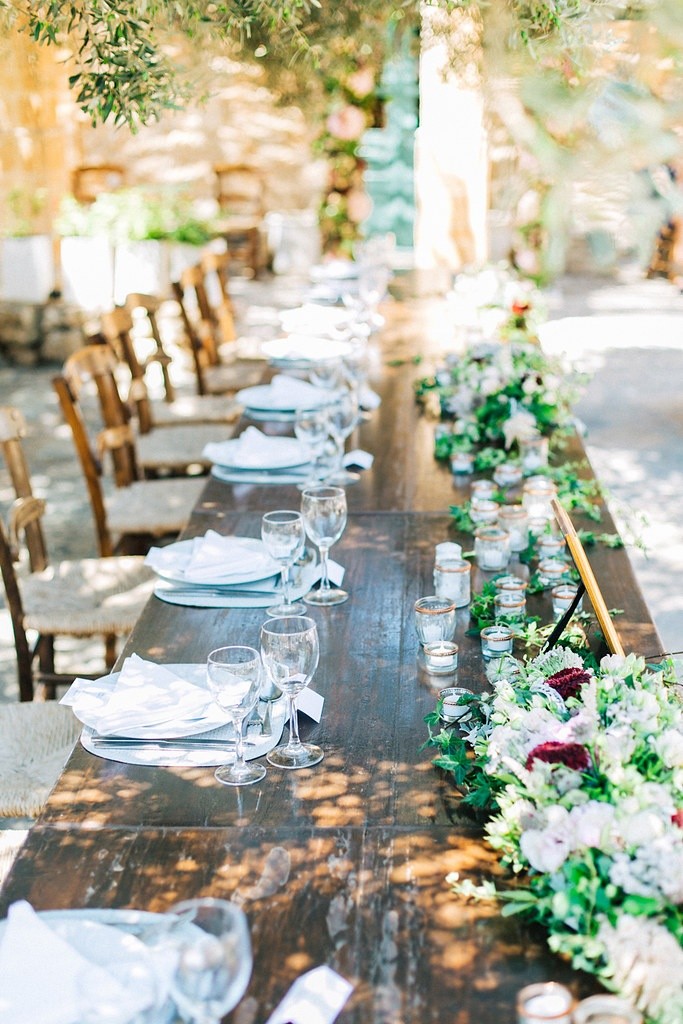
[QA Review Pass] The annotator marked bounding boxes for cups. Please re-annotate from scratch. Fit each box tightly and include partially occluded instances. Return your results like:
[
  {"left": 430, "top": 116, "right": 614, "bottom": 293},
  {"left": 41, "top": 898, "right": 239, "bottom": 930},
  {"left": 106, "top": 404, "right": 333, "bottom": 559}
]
[
  {"left": 514, "top": 982, "right": 645, "bottom": 1024},
  {"left": 413, "top": 430, "right": 584, "bottom": 728},
  {"left": 156, "top": 896, "right": 253, "bottom": 1024}
]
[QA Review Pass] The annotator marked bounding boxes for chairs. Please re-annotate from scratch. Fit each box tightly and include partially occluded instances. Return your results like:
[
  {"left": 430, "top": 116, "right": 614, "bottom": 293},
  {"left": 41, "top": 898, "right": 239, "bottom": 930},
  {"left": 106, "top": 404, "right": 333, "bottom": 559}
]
[{"left": 0, "top": 238, "right": 273, "bottom": 887}]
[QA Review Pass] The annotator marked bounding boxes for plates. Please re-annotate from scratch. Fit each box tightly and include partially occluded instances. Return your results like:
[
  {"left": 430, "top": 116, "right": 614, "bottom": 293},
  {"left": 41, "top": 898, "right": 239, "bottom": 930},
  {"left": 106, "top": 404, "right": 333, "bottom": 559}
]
[
  {"left": 1, "top": 909, "right": 180, "bottom": 1024},
  {"left": 145, "top": 535, "right": 289, "bottom": 586},
  {"left": 61, "top": 663, "right": 256, "bottom": 740},
  {"left": 202, "top": 252, "right": 356, "bottom": 468}
]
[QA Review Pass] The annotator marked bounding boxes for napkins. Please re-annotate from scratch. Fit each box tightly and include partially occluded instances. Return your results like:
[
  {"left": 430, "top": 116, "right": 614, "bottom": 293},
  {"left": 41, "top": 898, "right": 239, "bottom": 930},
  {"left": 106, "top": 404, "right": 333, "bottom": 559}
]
[
  {"left": 267, "top": 373, "right": 327, "bottom": 408},
  {"left": 92, "top": 651, "right": 217, "bottom": 738},
  {"left": 182, "top": 528, "right": 278, "bottom": 578},
  {"left": 0, "top": 897, "right": 168, "bottom": 1024},
  {"left": 228, "top": 426, "right": 309, "bottom": 467}
]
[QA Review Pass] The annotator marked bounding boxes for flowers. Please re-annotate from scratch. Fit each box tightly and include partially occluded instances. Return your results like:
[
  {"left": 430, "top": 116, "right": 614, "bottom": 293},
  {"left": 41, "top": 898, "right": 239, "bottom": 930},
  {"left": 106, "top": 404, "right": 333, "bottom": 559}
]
[{"left": 414, "top": 300, "right": 683, "bottom": 1024}]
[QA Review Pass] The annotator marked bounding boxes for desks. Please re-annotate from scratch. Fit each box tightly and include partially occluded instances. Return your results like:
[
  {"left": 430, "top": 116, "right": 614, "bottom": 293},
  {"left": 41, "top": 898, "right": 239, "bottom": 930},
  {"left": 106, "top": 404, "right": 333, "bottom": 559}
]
[
  {"left": 192, "top": 268, "right": 608, "bottom": 509},
  {"left": 0, "top": 824, "right": 615, "bottom": 1024},
  {"left": 40, "top": 508, "right": 667, "bottom": 823}
]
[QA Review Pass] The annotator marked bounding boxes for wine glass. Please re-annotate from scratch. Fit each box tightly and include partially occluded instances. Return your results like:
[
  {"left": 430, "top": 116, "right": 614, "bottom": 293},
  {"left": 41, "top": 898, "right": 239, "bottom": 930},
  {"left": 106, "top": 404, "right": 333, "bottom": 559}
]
[
  {"left": 260, "top": 615, "right": 324, "bottom": 769},
  {"left": 293, "top": 259, "right": 390, "bottom": 494},
  {"left": 206, "top": 646, "right": 267, "bottom": 785},
  {"left": 261, "top": 510, "right": 307, "bottom": 618},
  {"left": 301, "top": 486, "right": 349, "bottom": 606}
]
